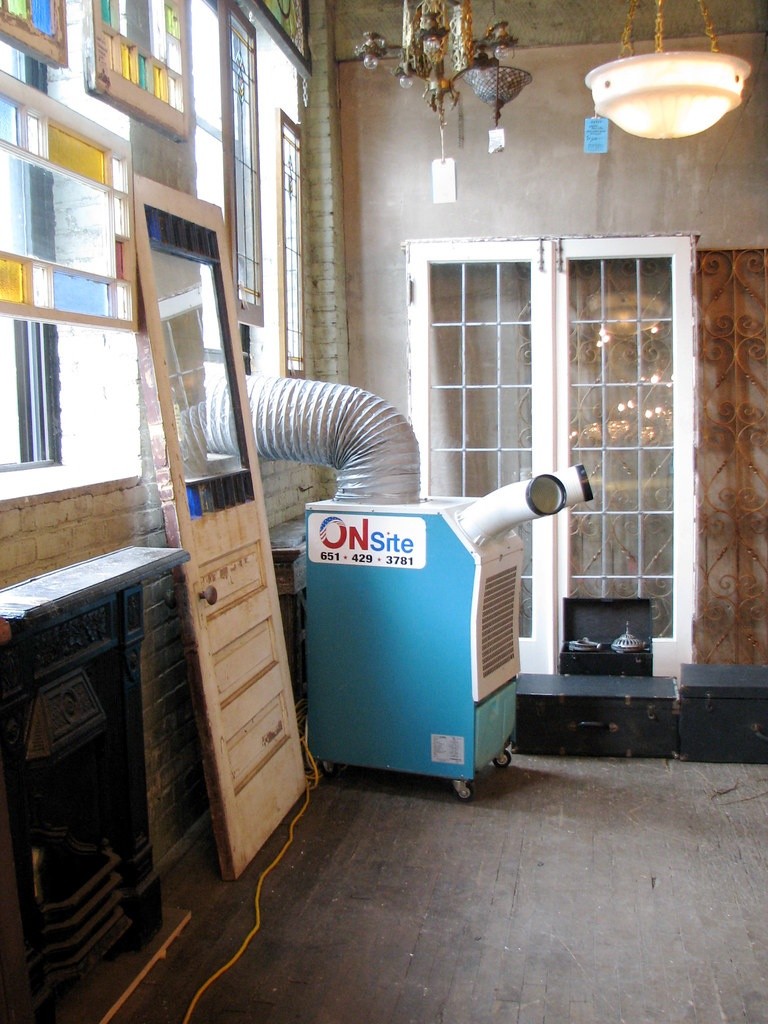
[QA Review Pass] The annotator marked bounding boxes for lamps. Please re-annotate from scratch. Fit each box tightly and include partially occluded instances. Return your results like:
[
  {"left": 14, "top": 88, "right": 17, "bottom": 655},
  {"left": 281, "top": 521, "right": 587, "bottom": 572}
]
[
  {"left": 463, "top": 0, "right": 533, "bottom": 127},
  {"left": 584, "top": 0, "right": 751, "bottom": 140},
  {"left": 354, "top": 0, "right": 521, "bottom": 130}
]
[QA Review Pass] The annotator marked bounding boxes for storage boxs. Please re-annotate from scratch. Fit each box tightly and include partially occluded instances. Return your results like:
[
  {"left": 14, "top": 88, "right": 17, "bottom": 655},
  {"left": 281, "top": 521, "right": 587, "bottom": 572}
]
[
  {"left": 679, "top": 663, "right": 768, "bottom": 764},
  {"left": 559, "top": 596, "right": 653, "bottom": 677},
  {"left": 510, "top": 674, "right": 679, "bottom": 759}
]
